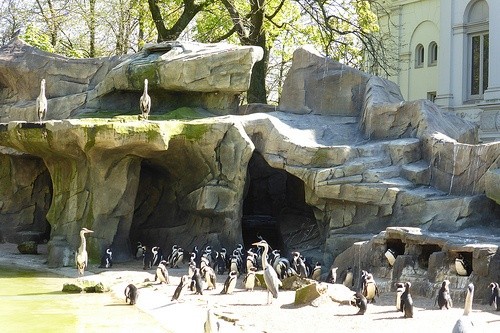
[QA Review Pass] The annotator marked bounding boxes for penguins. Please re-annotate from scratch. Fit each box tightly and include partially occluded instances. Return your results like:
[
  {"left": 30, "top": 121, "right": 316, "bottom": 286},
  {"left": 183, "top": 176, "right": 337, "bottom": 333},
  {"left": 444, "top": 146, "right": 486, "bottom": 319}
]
[
  {"left": 487, "top": 282, "right": 500, "bottom": 311},
  {"left": 125, "top": 284, "right": 139, "bottom": 305},
  {"left": 462, "top": 283, "right": 477, "bottom": 315},
  {"left": 402, "top": 282, "right": 413, "bottom": 318},
  {"left": 455, "top": 252, "right": 472, "bottom": 276},
  {"left": 381, "top": 240, "right": 397, "bottom": 266},
  {"left": 135, "top": 234, "right": 379, "bottom": 316},
  {"left": 434, "top": 279, "right": 452, "bottom": 309},
  {"left": 451, "top": 319, "right": 465, "bottom": 333},
  {"left": 394, "top": 282, "right": 405, "bottom": 312},
  {"left": 98, "top": 248, "right": 113, "bottom": 268}
]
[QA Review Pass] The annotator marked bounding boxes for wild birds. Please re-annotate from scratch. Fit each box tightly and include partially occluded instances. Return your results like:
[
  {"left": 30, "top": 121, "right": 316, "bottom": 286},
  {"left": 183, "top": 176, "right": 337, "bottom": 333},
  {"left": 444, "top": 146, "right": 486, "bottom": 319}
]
[
  {"left": 75, "top": 228, "right": 95, "bottom": 277},
  {"left": 36, "top": 78, "right": 47, "bottom": 124},
  {"left": 140, "top": 77, "right": 152, "bottom": 121}
]
